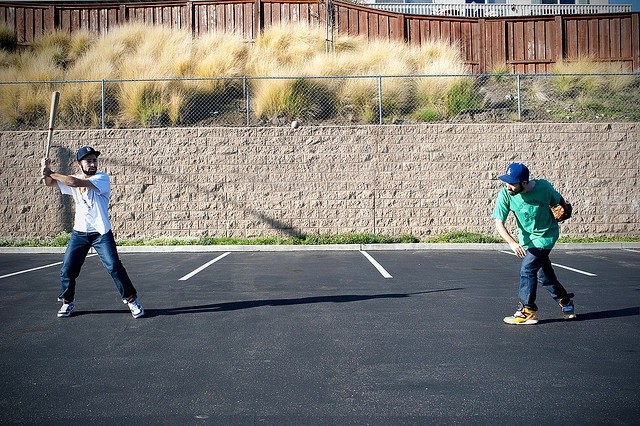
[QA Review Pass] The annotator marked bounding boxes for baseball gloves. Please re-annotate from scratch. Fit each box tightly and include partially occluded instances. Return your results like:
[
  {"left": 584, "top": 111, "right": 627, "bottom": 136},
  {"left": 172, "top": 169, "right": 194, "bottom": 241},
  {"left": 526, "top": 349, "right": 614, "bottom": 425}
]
[{"left": 551, "top": 204, "right": 572, "bottom": 222}]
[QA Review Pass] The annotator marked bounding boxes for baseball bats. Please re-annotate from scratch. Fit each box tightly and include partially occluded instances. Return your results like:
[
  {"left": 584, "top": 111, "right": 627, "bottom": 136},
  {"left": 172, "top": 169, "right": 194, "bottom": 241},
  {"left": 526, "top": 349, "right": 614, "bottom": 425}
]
[{"left": 43, "top": 91, "right": 61, "bottom": 179}]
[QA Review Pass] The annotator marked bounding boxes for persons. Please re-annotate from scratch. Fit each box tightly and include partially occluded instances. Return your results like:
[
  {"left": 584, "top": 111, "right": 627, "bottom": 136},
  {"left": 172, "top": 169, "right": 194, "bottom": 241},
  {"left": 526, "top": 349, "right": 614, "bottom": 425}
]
[
  {"left": 41, "top": 146, "right": 144, "bottom": 320},
  {"left": 491, "top": 162, "right": 577, "bottom": 326}
]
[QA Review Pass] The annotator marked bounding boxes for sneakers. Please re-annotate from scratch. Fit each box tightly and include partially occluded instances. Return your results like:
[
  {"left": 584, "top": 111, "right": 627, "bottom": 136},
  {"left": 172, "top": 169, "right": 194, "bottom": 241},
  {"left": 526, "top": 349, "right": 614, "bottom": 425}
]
[
  {"left": 561, "top": 304, "right": 577, "bottom": 320},
  {"left": 127, "top": 298, "right": 144, "bottom": 319},
  {"left": 503, "top": 307, "right": 539, "bottom": 325},
  {"left": 57, "top": 302, "right": 75, "bottom": 318}
]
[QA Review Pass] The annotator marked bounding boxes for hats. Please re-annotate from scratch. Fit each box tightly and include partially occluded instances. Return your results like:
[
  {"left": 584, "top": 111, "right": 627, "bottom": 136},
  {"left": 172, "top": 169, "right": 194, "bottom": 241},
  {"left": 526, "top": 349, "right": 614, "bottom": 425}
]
[
  {"left": 498, "top": 162, "right": 529, "bottom": 185},
  {"left": 77, "top": 146, "right": 101, "bottom": 160}
]
